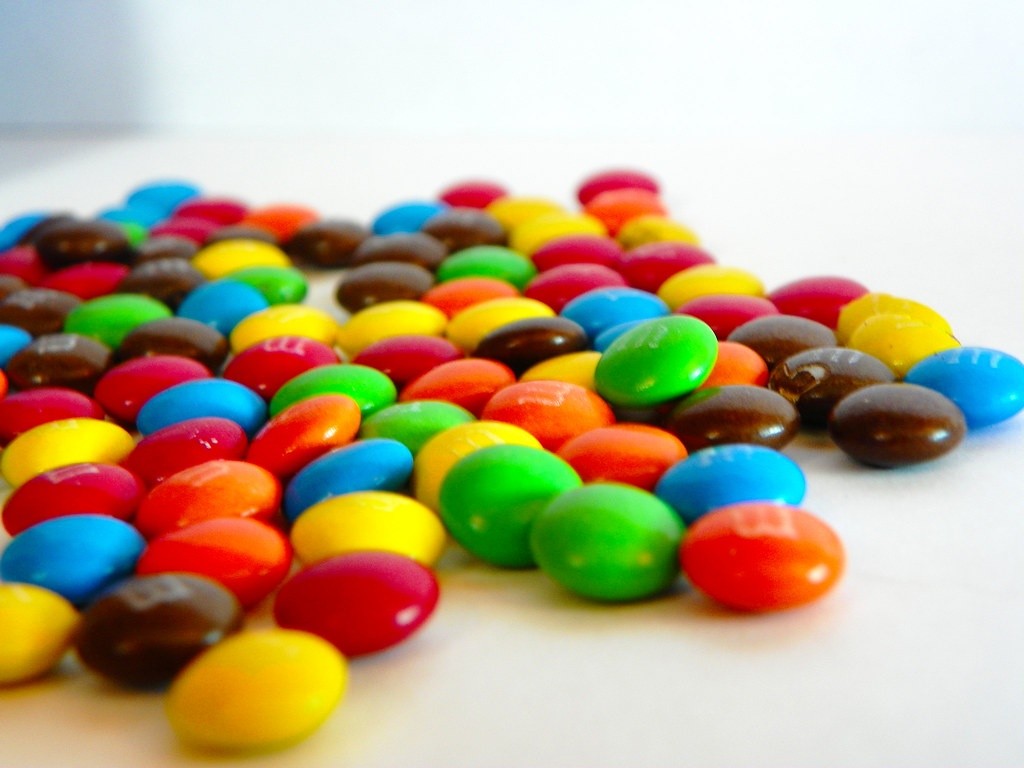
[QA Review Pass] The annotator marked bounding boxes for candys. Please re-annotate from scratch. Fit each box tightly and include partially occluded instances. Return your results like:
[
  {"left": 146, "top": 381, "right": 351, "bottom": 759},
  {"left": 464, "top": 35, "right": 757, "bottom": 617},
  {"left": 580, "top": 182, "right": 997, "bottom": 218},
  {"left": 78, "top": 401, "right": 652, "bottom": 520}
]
[{"left": 0, "top": 176, "right": 1023, "bottom": 750}]
